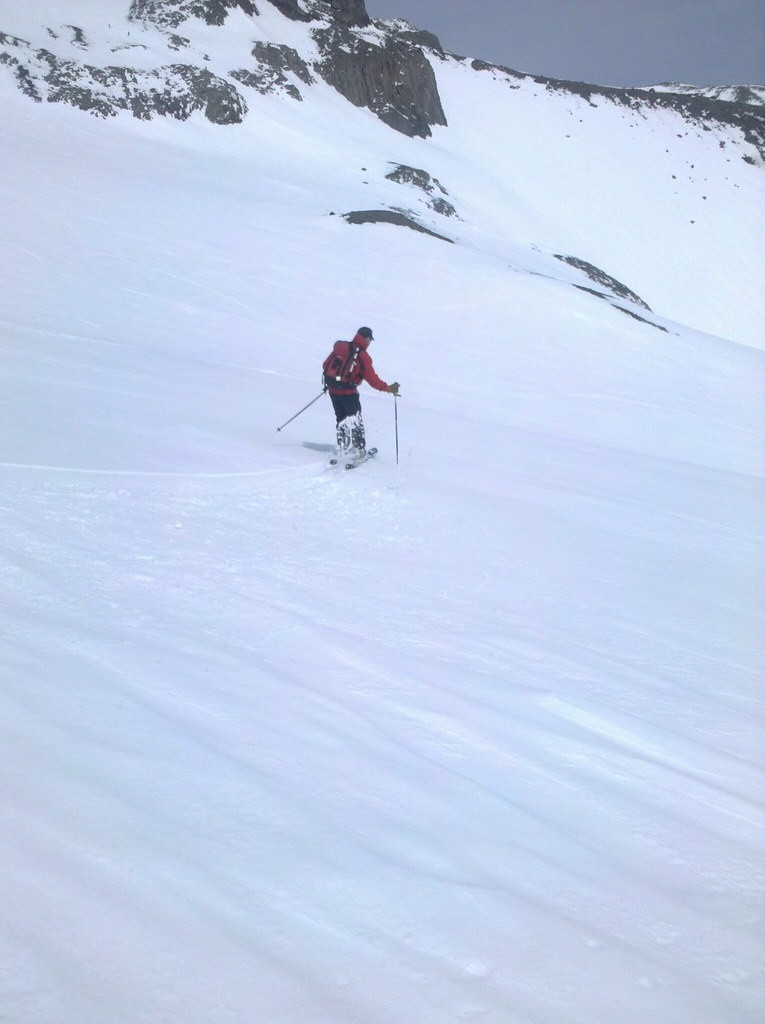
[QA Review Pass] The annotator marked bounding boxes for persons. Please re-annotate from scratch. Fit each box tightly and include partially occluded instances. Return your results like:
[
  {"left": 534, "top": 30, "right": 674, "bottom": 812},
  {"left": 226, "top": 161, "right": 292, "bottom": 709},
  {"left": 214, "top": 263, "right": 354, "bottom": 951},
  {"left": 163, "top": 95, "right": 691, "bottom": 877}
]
[{"left": 320, "top": 326, "right": 398, "bottom": 461}]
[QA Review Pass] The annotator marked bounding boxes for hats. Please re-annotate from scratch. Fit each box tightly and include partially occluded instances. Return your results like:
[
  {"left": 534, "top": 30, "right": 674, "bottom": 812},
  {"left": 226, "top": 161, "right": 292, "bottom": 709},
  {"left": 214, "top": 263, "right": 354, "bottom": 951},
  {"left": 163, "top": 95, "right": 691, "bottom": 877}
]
[{"left": 358, "top": 327, "right": 374, "bottom": 341}]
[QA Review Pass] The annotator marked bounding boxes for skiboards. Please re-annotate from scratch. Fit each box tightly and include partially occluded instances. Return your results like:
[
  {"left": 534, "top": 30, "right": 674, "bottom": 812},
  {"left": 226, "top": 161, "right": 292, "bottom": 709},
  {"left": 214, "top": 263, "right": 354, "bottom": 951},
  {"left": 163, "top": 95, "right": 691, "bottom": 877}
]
[
  {"left": 345, "top": 447, "right": 378, "bottom": 471},
  {"left": 330, "top": 459, "right": 341, "bottom": 465}
]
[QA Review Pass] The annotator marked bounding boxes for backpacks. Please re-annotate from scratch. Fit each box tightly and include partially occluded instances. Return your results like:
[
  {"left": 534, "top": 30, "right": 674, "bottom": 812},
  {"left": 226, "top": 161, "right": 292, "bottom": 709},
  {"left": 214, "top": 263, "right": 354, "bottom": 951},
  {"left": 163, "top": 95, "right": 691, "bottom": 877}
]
[{"left": 323, "top": 345, "right": 365, "bottom": 385}]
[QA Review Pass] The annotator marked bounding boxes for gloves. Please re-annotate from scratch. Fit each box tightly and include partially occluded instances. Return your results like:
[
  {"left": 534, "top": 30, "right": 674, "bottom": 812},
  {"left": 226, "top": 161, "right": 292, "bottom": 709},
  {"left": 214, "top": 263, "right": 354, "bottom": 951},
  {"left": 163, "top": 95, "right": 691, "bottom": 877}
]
[{"left": 388, "top": 383, "right": 400, "bottom": 393}]
